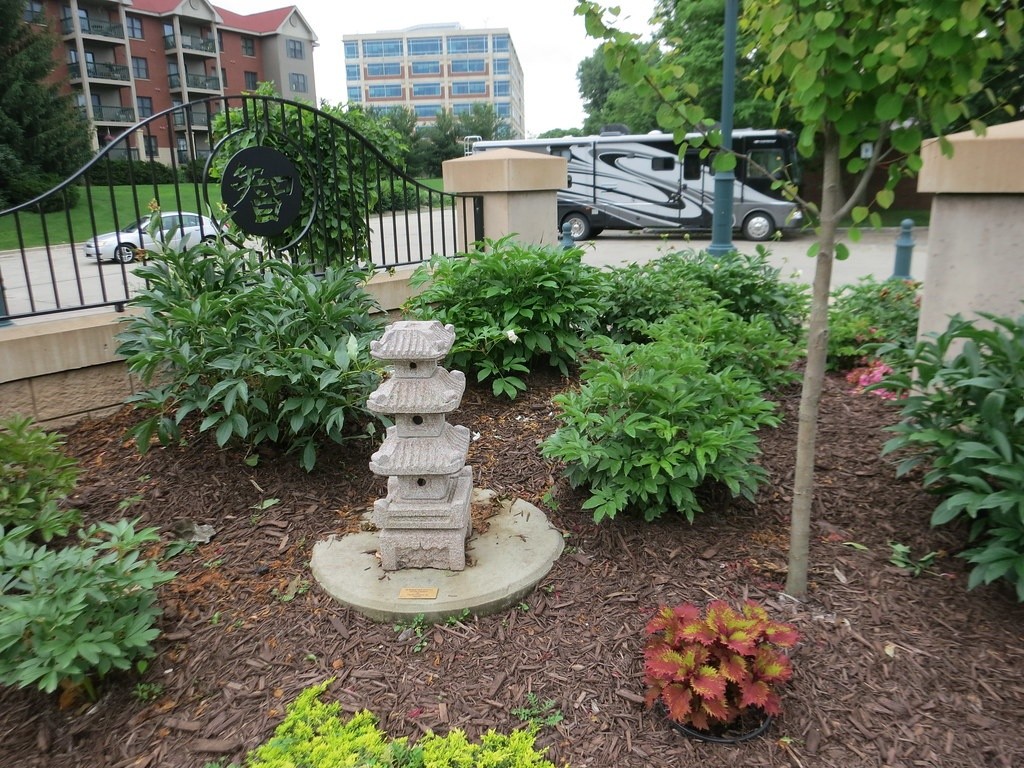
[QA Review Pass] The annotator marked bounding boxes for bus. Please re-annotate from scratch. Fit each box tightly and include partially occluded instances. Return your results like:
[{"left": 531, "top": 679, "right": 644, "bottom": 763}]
[{"left": 463, "top": 126, "right": 803, "bottom": 241}]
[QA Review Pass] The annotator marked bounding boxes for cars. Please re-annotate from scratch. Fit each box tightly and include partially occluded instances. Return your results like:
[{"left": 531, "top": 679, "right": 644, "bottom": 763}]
[{"left": 84, "top": 211, "right": 231, "bottom": 265}]
